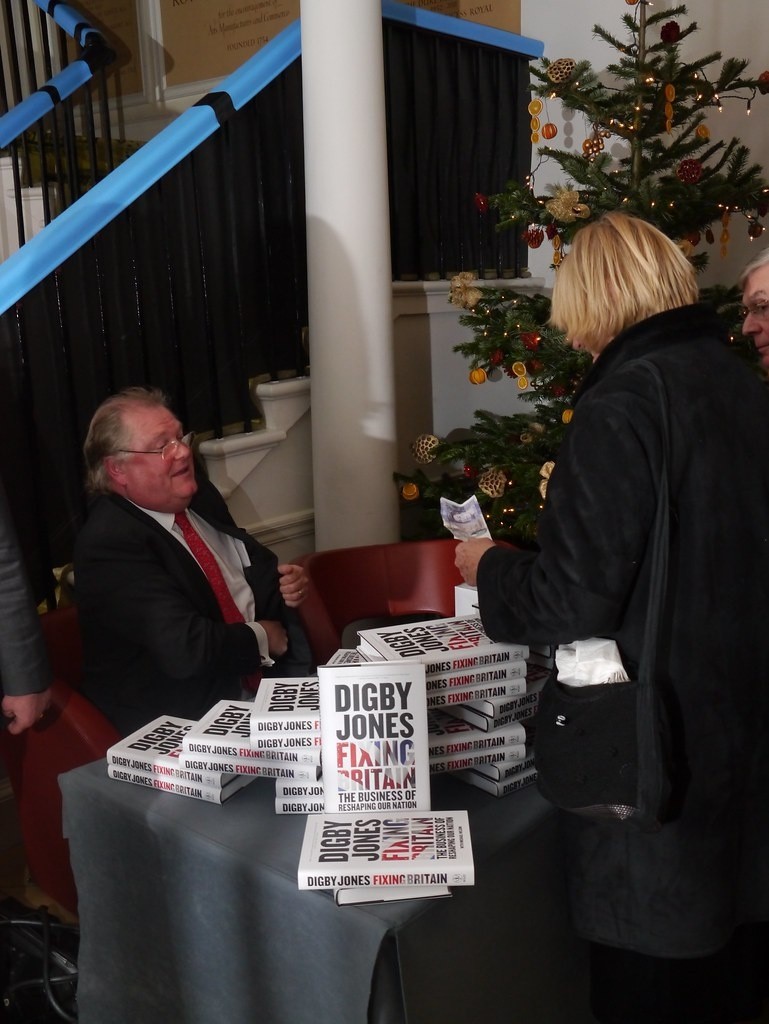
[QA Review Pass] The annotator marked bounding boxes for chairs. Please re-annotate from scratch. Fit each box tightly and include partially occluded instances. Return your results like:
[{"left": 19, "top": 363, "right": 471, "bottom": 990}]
[
  {"left": 17, "top": 608, "right": 120, "bottom": 920},
  {"left": 302, "top": 536, "right": 517, "bottom": 666}
]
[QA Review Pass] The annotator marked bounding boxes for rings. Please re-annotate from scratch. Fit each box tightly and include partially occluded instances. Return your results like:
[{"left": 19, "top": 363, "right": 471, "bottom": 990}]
[{"left": 298, "top": 590, "right": 304, "bottom": 596}]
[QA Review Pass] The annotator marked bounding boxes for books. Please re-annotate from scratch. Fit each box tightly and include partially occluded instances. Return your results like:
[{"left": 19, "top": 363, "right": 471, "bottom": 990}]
[{"left": 105, "top": 614, "right": 553, "bottom": 907}]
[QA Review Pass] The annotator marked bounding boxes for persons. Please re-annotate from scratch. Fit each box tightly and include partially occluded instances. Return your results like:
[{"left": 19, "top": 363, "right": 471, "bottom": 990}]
[
  {"left": 72, "top": 388, "right": 308, "bottom": 722},
  {"left": 737, "top": 245, "right": 769, "bottom": 368},
  {"left": 454, "top": 211, "right": 769, "bottom": 1024},
  {"left": 0, "top": 475, "right": 55, "bottom": 735}
]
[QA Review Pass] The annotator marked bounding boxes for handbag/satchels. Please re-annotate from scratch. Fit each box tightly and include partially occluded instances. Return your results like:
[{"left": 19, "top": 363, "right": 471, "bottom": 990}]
[{"left": 531, "top": 657, "right": 670, "bottom": 829}]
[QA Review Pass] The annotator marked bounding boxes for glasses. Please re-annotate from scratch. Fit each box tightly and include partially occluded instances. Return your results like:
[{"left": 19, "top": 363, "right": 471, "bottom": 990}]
[
  {"left": 120, "top": 431, "right": 195, "bottom": 461},
  {"left": 739, "top": 298, "right": 769, "bottom": 321}
]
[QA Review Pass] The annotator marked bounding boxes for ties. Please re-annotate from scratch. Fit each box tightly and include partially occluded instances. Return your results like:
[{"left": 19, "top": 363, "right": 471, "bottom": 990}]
[{"left": 174, "top": 512, "right": 265, "bottom": 692}]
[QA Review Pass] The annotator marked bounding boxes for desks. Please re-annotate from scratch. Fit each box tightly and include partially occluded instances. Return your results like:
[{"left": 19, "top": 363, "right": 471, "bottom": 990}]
[{"left": 57, "top": 753, "right": 593, "bottom": 1024}]
